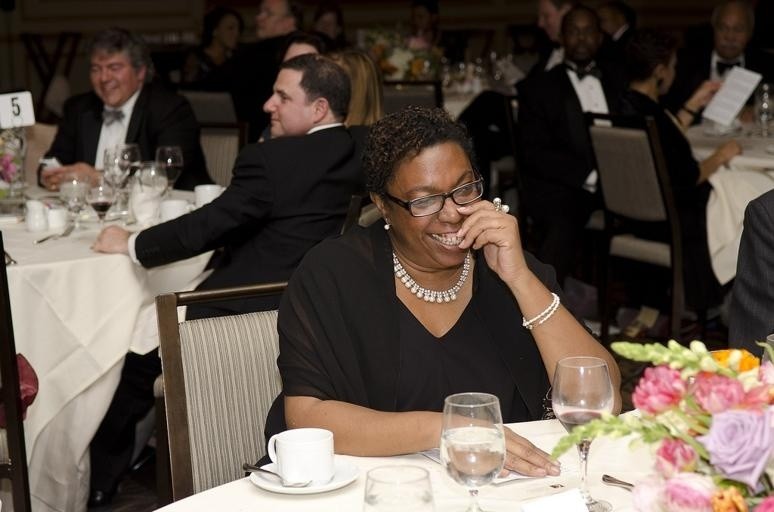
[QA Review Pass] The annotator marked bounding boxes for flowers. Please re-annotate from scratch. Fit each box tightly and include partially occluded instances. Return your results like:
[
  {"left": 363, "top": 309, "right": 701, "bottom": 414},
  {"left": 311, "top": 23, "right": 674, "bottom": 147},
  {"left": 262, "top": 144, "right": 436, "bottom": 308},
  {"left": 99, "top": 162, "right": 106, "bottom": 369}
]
[
  {"left": 555, "top": 334, "right": 774, "bottom": 512},
  {"left": 363, "top": 27, "right": 450, "bottom": 87}
]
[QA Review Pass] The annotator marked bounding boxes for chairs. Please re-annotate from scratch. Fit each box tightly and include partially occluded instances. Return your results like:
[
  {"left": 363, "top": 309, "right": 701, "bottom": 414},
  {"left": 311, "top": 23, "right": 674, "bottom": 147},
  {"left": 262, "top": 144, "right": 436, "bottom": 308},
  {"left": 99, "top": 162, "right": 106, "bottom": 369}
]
[
  {"left": 152, "top": 282, "right": 307, "bottom": 498},
  {"left": 583, "top": 109, "right": 711, "bottom": 351},
  {"left": 372, "top": 73, "right": 446, "bottom": 116}
]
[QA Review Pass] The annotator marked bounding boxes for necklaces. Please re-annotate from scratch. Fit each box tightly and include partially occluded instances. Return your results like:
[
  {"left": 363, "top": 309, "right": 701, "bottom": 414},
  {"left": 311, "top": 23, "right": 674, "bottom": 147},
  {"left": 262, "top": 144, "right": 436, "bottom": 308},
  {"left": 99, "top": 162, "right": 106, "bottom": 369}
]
[{"left": 392, "top": 250, "right": 475, "bottom": 304}]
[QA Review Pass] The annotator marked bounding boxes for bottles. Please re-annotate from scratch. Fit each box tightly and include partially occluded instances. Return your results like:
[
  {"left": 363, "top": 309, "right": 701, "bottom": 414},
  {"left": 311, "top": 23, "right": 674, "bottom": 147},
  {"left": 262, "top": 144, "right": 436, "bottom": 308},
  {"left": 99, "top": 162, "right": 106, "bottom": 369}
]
[{"left": 141, "top": 29, "right": 195, "bottom": 43}]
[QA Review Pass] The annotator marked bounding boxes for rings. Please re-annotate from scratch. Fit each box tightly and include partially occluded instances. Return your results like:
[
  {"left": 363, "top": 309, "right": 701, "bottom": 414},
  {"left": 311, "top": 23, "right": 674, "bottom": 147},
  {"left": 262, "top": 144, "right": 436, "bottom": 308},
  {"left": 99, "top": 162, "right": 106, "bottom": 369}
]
[{"left": 492, "top": 195, "right": 511, "bottom": 215}]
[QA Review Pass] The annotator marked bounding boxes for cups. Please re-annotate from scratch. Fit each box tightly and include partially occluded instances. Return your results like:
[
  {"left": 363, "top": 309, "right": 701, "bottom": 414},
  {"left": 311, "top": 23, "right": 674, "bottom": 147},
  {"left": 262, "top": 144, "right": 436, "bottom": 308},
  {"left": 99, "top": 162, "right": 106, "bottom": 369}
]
[
  {"left": 361, "top": 465, "right": 434, "bottom": 508},
  {"left": 20, "top": 200, "right": 66, "bottom": 231},
  {"left": 760, "top": 334, "right": 774, "bottom": 383},
  {"left": 129, "top": 182, "right": 228, "bottom": 227},
  {"left": 264, "top": 428, "right": 339, "bottom": 487}
]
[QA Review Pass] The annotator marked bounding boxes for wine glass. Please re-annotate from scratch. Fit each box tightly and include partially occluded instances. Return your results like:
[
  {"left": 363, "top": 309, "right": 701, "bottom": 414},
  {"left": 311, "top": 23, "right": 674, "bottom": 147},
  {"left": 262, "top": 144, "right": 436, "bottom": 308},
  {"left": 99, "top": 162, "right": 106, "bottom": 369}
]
[
  {"left": 751, "top": 82, "right": 774, "bottom": 139},
  {"left": 549, "top": 353, "right": 618, "bottom": 510},
  {"left": 437, "top": 393, "right": 504, "bottom": 512},
  {"left": 53, "top": 140, "right": 187, "bottom": 235},
  {"left": 440, "top": 55, "right": 489, "bottom": 99}
]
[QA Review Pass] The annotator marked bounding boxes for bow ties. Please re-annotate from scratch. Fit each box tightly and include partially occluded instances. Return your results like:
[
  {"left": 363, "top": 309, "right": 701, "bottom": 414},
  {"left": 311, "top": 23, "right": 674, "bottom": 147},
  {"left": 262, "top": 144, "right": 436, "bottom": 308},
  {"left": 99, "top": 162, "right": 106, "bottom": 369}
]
[
  {"left": 102, "top": 109, "right": 122, "bottom": 125},
  {"left": 576, "top": 65, "right": 600, "bottom": 80},
  {"left": 716, "top": 62, "right": 741, "bottom": 75}
]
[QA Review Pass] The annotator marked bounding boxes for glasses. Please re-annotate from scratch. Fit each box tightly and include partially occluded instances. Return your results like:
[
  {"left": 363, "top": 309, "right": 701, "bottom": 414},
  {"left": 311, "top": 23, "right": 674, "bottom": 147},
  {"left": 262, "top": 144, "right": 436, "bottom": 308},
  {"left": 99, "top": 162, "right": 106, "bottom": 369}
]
[{"left": 383, "top": 163, "right": 483, "bottom": 217}]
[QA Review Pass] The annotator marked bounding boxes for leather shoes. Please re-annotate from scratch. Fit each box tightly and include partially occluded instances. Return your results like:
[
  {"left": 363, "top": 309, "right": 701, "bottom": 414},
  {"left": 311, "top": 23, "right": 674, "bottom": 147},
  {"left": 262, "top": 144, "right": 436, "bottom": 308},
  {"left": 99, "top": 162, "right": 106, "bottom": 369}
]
[
  {"left": 86, "top": 463, "right": 130, "bottom": 507},
  {"left": 619, "top": 318, "right": 656, "bottom": 345},
  {"left": 667, "top": 320, "right": 730, "bottom": 347}
]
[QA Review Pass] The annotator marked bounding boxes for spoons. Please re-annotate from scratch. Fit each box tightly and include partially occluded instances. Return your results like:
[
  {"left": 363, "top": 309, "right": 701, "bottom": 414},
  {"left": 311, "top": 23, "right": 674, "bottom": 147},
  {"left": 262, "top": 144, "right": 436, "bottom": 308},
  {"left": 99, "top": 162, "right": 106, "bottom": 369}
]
[{"left": 242, "top": 464, "right": 312, "bottom": 489}]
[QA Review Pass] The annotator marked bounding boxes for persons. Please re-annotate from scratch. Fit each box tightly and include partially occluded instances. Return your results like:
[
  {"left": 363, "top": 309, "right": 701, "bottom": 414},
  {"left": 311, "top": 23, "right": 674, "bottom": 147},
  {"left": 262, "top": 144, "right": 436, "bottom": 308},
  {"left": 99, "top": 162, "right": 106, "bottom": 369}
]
[
  {"left": 731, "top": 187, "right": 774, "bottom": 358},
  {"left": 35, "top": 1, "right": 772, "bottom": 313},
  {"left": 88, "top": 51, "right": 370, "bottom": 512},
  {"left": 248, "top": 107, "right": 622, "bottom": 478}
]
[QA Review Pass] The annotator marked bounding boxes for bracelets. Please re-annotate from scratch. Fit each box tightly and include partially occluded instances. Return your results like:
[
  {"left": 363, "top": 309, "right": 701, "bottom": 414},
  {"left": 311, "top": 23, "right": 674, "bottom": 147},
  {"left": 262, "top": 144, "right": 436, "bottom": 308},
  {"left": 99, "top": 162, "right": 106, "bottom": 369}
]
[{"left": 519, "top": 292, "right": 561, "bottom": 328}]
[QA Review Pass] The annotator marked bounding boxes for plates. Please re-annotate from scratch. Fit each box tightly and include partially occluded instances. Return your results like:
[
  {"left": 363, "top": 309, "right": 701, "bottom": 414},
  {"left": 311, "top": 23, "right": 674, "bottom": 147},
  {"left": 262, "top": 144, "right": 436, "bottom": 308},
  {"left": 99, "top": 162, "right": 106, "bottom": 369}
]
[
  {"left": 247, "top": 461, "right": 368, "bottom": 498},
  {"left": 699, "top": 125, "right": 740, "bottom": 141}
]
